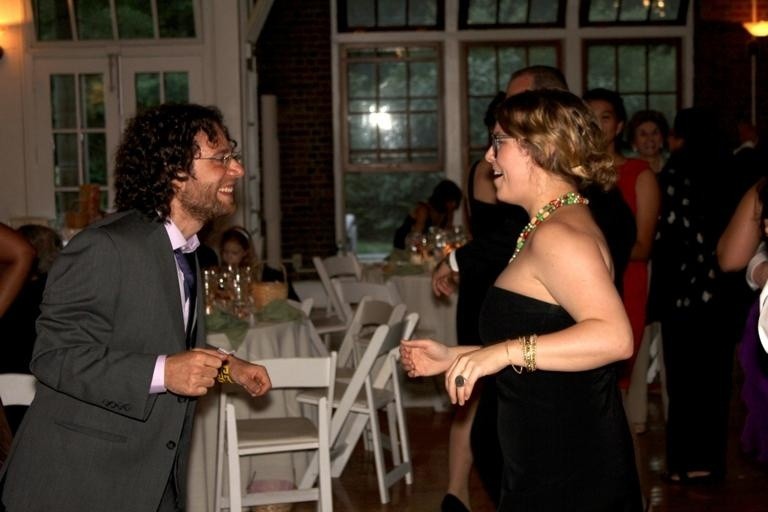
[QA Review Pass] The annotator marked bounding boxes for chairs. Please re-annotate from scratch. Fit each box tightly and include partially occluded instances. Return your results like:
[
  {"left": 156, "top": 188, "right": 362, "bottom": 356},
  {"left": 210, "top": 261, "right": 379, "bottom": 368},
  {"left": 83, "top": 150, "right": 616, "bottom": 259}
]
[{"left": 184, "top": 250, "right": 466, "bottom": 511}]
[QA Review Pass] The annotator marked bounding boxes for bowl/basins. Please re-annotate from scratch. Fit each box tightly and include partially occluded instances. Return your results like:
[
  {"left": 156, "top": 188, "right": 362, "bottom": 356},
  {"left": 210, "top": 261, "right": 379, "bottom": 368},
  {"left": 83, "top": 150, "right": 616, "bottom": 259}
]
[{"left": 66, "top": 211, "right": 89, "bottom": 229}]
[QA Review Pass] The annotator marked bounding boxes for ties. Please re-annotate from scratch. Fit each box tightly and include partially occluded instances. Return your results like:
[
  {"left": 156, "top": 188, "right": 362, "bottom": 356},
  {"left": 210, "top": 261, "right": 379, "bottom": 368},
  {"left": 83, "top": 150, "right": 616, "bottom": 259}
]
[{"left": 169, "top": 251, "right": 200, "bottom": 344}]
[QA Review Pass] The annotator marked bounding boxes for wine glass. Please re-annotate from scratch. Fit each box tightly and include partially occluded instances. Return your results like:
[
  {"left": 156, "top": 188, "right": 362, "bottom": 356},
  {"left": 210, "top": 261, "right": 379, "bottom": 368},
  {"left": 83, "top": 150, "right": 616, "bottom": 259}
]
[
  {"left": 202, "top": 265, "right": 254, "bottom": 318},
  {"left": 406, "top": 223, "right": 467, "bottom": 265}
]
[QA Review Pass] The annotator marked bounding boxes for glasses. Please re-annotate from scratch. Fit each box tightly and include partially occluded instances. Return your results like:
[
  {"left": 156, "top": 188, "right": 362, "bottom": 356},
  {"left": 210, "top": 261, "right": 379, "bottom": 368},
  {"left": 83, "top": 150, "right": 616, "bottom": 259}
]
[
  {"left": 487, "top": 127, "right": 516, "bottom": 158},
  {"left": 194, "top": 147, "right": 244, "bottom": 169}
]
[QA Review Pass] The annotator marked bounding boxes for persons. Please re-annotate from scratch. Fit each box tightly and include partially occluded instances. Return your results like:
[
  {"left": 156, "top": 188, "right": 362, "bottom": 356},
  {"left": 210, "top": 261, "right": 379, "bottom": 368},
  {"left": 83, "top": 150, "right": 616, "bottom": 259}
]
[
  {"left": 432, "top": 64, "right": 767, "bottom": 512},
  {"left": 1, "top": 222, "right": 37, "bottom": 317},
  {"left": 220, "top": 225, "right": 253, "bottom": 269},
  {"left": 1, "top": 103, "right": 272, "bottom": 512},
  {"left": 398, "top": 87, "right": 646, "bottom": 511},
  {"left": 393, "top": 180, "right": 462, "bottom": 250},
  {"left": 1, "top": 225, "right": 63, "bottom": 434}
]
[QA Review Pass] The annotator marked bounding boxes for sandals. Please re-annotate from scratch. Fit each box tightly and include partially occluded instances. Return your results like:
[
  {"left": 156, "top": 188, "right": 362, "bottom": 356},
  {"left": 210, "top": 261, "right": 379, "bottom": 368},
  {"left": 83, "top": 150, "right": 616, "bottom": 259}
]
[{"left": 659, "top": 465, "right": 722, "bottom": 495}]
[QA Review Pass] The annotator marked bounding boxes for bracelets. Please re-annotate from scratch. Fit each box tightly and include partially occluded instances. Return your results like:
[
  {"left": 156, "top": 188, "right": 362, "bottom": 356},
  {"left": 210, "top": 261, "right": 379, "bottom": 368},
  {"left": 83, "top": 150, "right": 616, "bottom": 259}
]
[{"left": 213, "top": 354, "right": 233, "bottom": 385}]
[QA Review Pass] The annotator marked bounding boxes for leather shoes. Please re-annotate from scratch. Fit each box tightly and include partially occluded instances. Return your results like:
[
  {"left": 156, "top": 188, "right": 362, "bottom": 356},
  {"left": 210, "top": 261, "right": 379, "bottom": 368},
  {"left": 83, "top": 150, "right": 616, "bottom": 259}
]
[{"left": 441, "top": 494, "right": 471, "bottom": 511}]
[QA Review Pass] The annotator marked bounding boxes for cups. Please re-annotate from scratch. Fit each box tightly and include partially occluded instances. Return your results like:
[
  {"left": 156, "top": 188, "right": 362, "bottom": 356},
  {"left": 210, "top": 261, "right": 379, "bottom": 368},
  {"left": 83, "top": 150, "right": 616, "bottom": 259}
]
[{"left": 78, "top": 182, "right": 100, "bottom": 224}]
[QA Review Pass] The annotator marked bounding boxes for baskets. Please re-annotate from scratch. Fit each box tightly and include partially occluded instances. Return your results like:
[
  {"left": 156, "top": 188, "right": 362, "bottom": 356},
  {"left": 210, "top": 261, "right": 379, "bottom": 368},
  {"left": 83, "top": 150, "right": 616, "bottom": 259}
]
[{"left": 245, "top": 262, "right": 289, "bottom": 311}]
